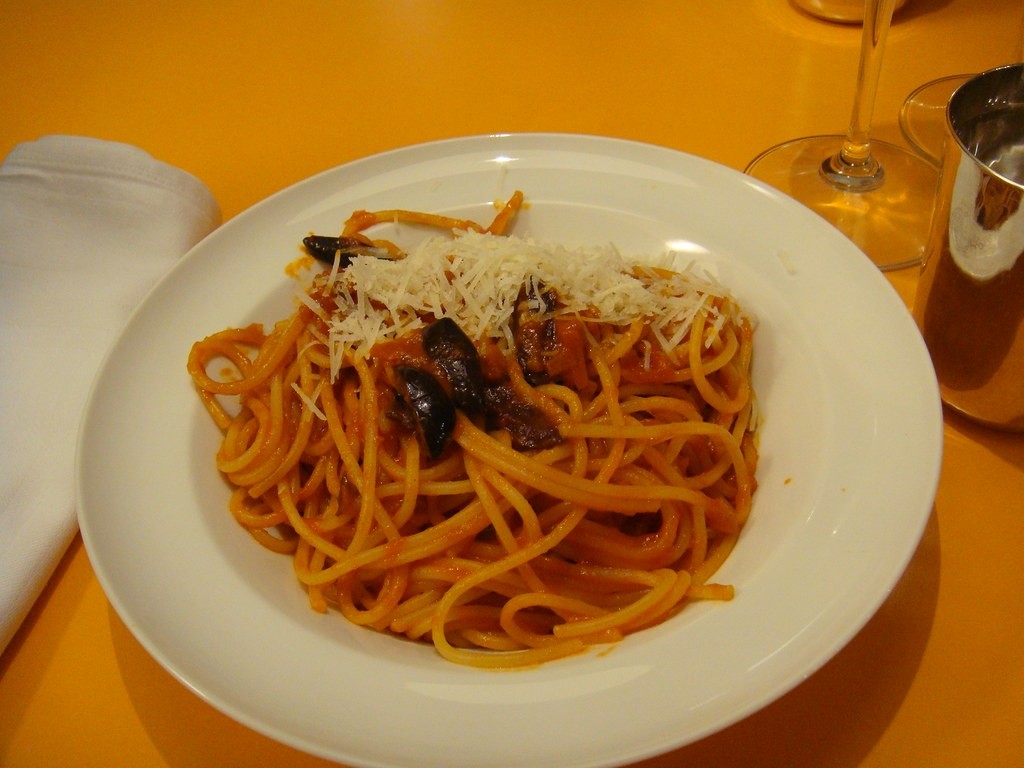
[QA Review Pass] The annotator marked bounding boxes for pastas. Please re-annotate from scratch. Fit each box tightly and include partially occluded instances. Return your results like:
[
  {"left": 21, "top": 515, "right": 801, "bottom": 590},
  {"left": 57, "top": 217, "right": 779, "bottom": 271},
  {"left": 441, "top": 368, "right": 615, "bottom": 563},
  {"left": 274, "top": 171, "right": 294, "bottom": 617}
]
[{"left": 186, "top": 188, "right": 756, "bottom": 670}]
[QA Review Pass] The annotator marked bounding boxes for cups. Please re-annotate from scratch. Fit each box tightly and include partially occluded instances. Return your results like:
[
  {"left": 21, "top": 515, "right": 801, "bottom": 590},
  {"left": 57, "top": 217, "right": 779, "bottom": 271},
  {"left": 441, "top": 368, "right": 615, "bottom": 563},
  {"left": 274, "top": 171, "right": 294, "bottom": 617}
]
[
  {"left": 913, "top": 64, "right": 1024, "bottom": 435},
  {"left": 792, "top": 0, "right": 918, "bottom": 27}
]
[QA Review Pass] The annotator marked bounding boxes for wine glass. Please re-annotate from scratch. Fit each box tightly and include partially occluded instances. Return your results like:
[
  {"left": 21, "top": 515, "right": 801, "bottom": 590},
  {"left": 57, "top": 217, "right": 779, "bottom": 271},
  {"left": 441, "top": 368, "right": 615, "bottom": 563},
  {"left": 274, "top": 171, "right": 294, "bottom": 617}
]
[
  {"left": 900, "top": 72, "right": 977, "bottom": 163},
  {"left": 744, "top": 0, "right": 940, "bottom": 273}
]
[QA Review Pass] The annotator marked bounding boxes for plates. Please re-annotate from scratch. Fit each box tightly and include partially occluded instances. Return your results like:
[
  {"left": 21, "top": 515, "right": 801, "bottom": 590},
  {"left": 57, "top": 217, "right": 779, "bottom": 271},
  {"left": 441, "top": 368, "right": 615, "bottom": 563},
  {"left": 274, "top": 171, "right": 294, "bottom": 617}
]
[{"left": 75, "top": 130, "right": 945, "bottom": 768}]
